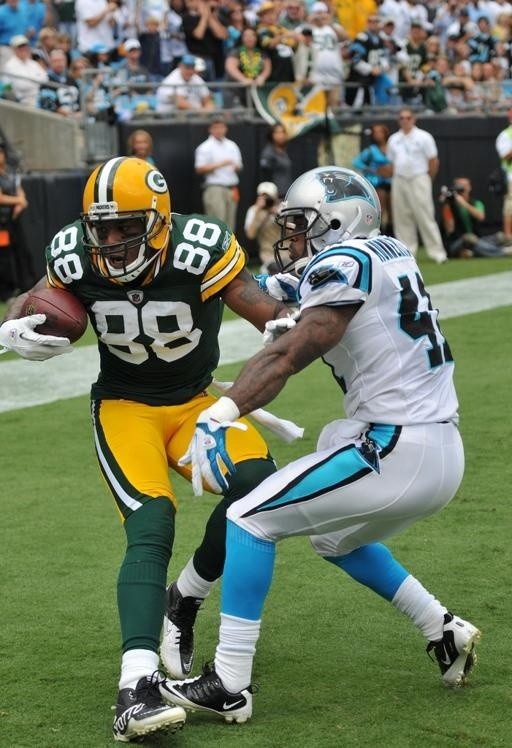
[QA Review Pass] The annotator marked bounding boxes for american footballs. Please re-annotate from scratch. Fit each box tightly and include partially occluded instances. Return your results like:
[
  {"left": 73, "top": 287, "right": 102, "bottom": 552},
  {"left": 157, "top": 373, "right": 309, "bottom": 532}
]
[{"left": 20, "top": 288, "right": 88, "bottom": 344}]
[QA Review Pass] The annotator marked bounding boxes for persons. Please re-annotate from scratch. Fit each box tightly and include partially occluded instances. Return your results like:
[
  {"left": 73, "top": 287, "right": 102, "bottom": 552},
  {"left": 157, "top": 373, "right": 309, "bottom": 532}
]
[
  {"left": 1, "top": 154, "right": 294, "bottom": 743},
  {"left": 160, "top": 166, "right": 483, "bottom": 724},
  {"left": 1, "top": 1, "right": 510, "bottom": 306}
]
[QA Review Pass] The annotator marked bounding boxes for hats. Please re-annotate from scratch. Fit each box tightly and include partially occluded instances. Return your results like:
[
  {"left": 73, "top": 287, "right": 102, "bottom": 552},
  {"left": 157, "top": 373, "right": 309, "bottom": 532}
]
[
  {"left": 123, "top": 37, "right": 142, "bottom": 52},
  {"left": 8, "top": 34, "right": 30, "bottom": 47},
  {"left": 181, "top": 55, "right": 196, "bottom": 67},
  {"left": 256, "top": 1, "right": 275, "bottom": 16},
  {"left": 90, "top": 42, "right": 110, "bottom": 56}
]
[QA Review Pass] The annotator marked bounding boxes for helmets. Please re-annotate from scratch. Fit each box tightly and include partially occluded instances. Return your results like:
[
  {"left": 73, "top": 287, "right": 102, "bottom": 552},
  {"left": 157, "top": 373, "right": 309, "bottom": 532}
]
[
  {"left": 272, "top": 165, "right": 381, "bottom": 274},
  {"left": 79, "top": 157, "right": 175, "bottom": 290}
]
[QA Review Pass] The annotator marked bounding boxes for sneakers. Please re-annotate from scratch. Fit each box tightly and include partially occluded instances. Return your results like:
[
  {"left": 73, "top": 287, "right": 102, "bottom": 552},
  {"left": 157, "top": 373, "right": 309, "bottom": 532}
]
[
  {"left": 112, "top": 669, "right": 187, "bottom": 743},
  {"left": 425, "top": 611, "right": 482, "bottom": 689},
  {"left": 160, "top": 581, "right": 206, "bottom": 680},
  {"left": 159, "top": 658, "right": 260, "bottom": 724}
]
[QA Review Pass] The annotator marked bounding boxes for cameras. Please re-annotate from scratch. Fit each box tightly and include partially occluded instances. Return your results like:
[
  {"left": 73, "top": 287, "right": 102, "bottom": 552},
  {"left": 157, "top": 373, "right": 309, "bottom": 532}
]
[{"left": 445, "top": 184, "right": 464, "bottom": 204}]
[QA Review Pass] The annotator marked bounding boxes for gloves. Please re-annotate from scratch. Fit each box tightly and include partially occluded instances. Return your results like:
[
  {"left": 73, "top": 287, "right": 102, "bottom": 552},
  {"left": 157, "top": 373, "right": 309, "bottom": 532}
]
[
  {"left": 262, "top": 305, "right": 297, "bottom": 348},
  {"left": 177, "top": 395, "right": 248, "bottom": 498},
  {"left": 0, "top": 313, "right": 76, "bottom": 363}
]
